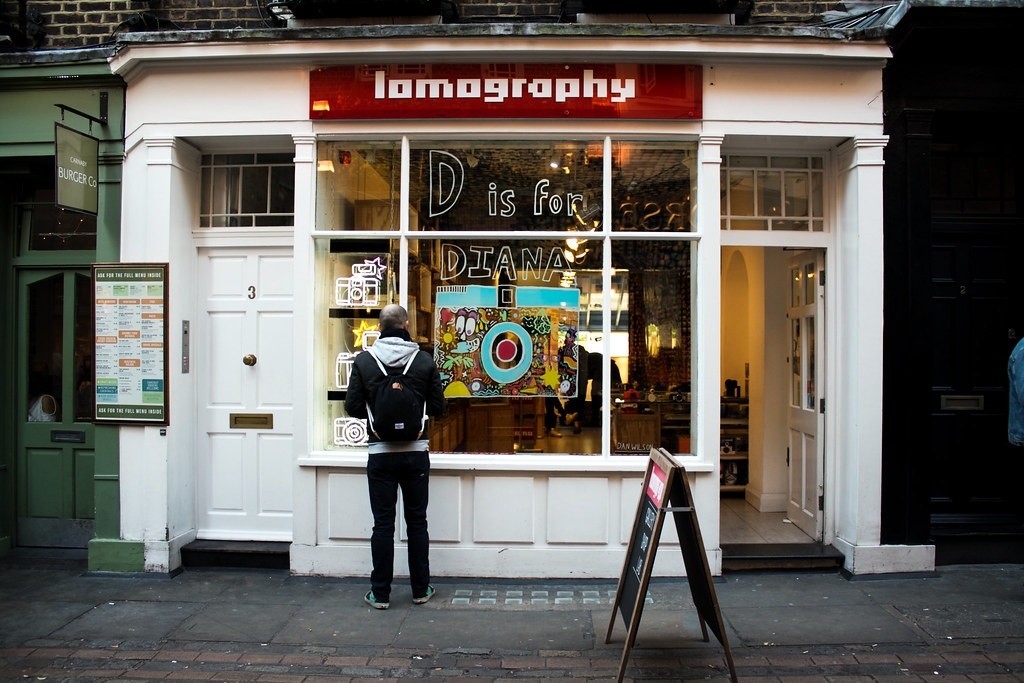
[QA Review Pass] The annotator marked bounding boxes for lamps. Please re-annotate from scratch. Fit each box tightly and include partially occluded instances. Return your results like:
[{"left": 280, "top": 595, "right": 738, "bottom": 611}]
[{"left": 266, "top": 0, "right": 298, "bottom": 22}]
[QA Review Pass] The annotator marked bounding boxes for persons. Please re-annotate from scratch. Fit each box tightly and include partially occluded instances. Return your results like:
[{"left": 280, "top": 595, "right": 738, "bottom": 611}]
[
  {"left": 344, "top": 304, "right": 448, "bottom": 609},
  {"left": 546, "top": 329, "right": 622, "bottom": 437}
]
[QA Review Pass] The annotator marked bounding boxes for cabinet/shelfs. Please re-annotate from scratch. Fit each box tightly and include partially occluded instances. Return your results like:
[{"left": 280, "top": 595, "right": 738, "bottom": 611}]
[
  {"left": 354, "top": 199, "right": 544, "bottom": 452},
  {"left": 663, "top": 426, "right": 749, "bottom": 493}
]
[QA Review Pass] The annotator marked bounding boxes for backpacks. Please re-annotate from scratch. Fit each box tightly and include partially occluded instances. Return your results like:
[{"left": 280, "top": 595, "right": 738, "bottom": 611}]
[{"left": 366, "top": 347, "right": 429, "bottom": 442}]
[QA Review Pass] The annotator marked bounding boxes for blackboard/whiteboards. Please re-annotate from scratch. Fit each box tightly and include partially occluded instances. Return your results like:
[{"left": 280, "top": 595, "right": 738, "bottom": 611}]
[{"left": 615, "top": 448, "right": 673, "bottom": 633}]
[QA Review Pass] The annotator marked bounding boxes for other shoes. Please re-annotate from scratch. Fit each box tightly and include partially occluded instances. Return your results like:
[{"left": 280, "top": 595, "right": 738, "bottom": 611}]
[
  {"left": 547, "top": 428, "right": 562, "bottom": 437},
  {"left": 565, "top": 412, "right": 579, "bottom": 425}
]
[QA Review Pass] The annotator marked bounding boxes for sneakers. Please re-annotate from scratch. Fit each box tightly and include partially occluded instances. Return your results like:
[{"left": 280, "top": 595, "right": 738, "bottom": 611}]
[
  {"left": 364, "top": 590, "right": 389, "bottom": 610},
  {"left": 411, "top": 583, "right": 435, "bottom": 604}
]
